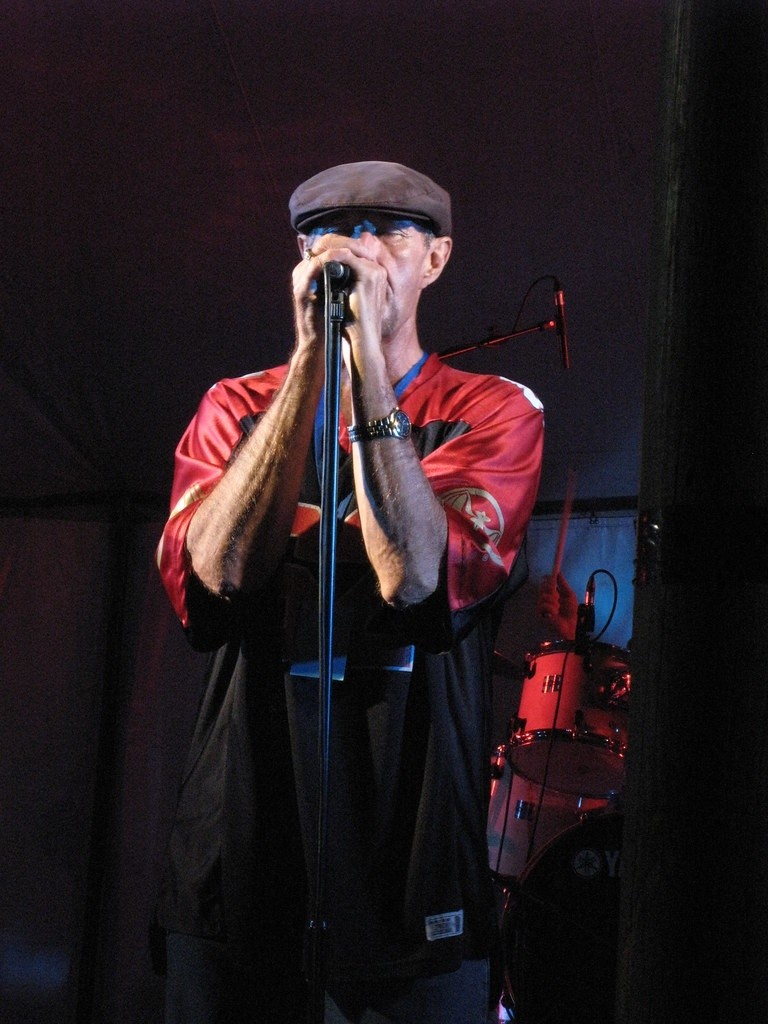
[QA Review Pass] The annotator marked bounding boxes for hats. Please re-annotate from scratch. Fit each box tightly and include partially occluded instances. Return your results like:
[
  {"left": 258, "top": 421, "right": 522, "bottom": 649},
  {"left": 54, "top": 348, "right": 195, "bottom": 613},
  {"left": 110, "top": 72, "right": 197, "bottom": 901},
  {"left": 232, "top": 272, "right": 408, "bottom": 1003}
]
[{"left": 284, "top": 160, "right": 455, "bottom": 245}]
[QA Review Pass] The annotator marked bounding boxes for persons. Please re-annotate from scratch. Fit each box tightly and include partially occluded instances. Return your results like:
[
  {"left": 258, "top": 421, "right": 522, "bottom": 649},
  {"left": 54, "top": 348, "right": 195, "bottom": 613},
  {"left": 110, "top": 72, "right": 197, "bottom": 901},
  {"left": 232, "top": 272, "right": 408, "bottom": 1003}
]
[
  {"left": 538, "top": 572, "right": 579, "bottom": 641},
  {"left": 151, "top": 160, "right": 546, "bottom": 1024}
]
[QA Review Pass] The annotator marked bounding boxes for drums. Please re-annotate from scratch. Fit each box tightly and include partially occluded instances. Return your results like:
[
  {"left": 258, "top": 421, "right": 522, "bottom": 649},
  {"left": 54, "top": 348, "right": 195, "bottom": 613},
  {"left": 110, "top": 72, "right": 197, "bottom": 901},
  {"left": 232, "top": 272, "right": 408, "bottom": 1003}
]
[
  {"left": 506, "top": 636, "right": 634, "bottom": 799},
  {"left": 469, "top": 741, "right": 609, "bottom": 881},
  {"left": 496, "top": 807, "right": 622, "bottom": 1024}
]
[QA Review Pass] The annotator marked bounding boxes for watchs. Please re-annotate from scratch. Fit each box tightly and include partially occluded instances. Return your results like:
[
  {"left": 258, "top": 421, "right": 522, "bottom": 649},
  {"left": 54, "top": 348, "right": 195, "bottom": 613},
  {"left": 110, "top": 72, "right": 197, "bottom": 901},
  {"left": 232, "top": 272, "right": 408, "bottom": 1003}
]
[{"left": 347, "top": 410, "right": 412, "bottom": 442}]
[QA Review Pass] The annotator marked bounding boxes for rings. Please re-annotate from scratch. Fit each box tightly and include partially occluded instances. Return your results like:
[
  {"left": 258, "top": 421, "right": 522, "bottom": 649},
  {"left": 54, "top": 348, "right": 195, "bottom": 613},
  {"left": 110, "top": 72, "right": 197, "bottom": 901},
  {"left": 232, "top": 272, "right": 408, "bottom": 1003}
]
[{"left": 303, "top": 247, "right": 317, "bottom": 260}]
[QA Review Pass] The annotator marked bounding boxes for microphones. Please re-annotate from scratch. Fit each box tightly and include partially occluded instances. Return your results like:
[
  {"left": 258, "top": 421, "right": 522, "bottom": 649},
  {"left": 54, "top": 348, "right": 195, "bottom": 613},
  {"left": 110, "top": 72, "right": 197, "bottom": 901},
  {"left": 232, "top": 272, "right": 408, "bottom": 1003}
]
[
  {"left": 317, "top": 261, "right": 354, "bottom": 290},
  {"left": 553, "top": 281, "right": 570, "bottom": 368},
  {"left": 583, "top": 576, "right": 597, "bottom": 632}
]
